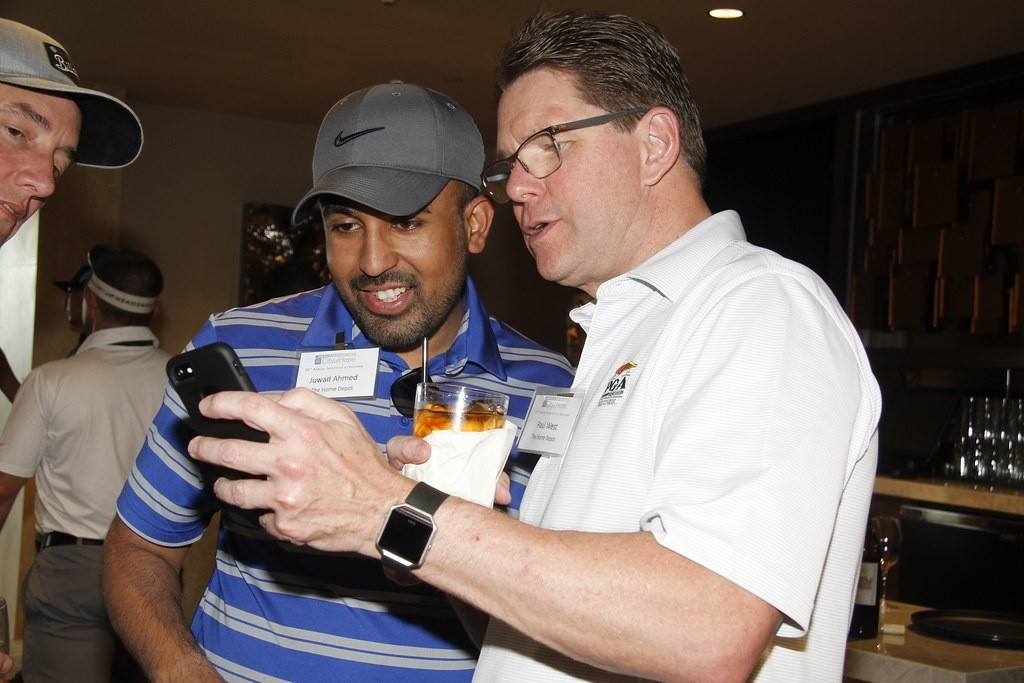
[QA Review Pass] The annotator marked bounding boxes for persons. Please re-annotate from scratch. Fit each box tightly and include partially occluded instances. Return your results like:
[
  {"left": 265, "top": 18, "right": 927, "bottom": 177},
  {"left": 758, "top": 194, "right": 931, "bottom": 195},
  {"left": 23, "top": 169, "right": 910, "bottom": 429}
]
[
  {"left": 1, "top": 18, "right": 88, "bottom": 250},
  {"left": 189, "top": 14, "right": 883, "bottom": 682},
  {"left": 102, "top": 81, "right": 574, "bottom": 683},
  {"left": 0, "top": 246, "right": 175, "bottom": 682}
]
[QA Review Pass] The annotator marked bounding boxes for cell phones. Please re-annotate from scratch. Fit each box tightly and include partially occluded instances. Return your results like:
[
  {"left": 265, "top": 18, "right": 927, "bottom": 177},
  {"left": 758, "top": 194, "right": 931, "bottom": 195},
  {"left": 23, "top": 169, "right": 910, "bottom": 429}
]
[{"left": 165, "top": 342, "right": 272, "bottom": 512}]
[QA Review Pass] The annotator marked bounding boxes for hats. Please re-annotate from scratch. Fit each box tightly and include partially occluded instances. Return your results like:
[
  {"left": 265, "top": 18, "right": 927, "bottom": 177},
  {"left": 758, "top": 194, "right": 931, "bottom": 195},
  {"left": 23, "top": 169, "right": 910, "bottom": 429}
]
[
  {"left": 0, "top": 18, "right": 143, "bottom": 169},
  {"left": 292, "top": 80, "right": 484, "bottom": 226},
  {"left": 52, "top": 263, "right": 91, "bottom": 292}
]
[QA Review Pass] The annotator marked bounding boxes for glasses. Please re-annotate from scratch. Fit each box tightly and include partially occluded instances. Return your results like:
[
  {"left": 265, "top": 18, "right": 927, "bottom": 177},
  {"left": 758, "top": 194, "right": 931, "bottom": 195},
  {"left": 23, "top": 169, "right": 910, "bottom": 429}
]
[{"left": 481, "top": 109, "right": 647, "bottom": 205}]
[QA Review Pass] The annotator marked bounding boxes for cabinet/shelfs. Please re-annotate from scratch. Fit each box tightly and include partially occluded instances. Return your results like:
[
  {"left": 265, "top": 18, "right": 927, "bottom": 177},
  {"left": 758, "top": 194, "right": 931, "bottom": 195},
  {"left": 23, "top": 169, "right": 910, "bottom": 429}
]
[{"left": 840, "top": 475, "right": 1023, "bottom": 683}]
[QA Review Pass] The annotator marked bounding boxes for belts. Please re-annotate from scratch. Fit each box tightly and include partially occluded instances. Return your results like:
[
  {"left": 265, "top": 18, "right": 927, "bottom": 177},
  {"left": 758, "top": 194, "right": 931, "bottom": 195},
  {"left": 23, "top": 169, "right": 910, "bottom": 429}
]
[{"left": 43, "top": 532, "right": 103, "bottom": 548}]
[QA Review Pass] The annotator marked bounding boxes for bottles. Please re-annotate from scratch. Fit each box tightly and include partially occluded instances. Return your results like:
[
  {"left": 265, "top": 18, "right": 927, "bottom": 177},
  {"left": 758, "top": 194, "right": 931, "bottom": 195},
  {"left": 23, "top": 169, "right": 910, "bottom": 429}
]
[
  {"left": 953, "top": 390, "right": 1024, "bottom": 490},
  {"left": 849, "top": 508, "right": 884, "bottom": 641}
]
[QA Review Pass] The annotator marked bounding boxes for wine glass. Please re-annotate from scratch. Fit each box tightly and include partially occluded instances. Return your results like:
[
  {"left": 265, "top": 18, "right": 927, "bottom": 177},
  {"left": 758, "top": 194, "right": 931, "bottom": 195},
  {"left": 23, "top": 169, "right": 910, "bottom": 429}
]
[{"left": 870, "top": 518, "right": 897, "bottom": 634}]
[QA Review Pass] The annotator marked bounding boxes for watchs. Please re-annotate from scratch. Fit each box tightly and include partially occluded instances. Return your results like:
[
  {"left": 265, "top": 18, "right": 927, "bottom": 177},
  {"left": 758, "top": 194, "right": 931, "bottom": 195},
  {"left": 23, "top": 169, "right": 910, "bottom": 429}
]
[{"left": 376, "top": 481, "right": 452, "bottom": 578}]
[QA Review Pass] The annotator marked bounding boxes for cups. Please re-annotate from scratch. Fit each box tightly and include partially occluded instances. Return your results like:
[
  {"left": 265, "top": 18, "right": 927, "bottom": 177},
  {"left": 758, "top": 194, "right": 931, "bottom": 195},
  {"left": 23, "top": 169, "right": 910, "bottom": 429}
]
[{"left": 412, "top": 382, "right": 510, "bottom": 440}]
